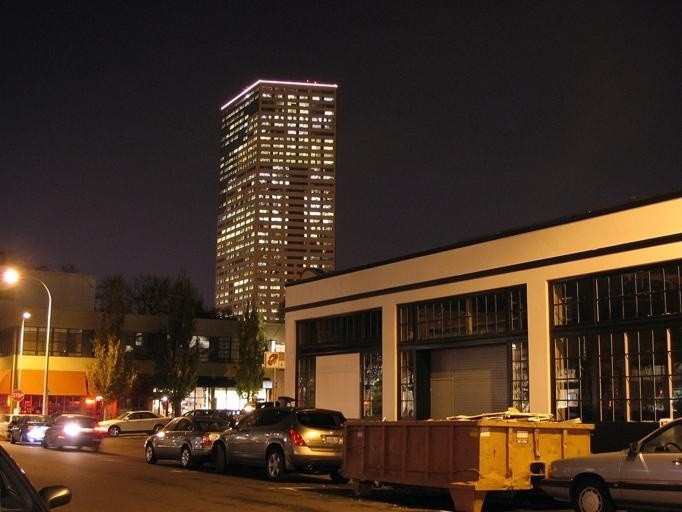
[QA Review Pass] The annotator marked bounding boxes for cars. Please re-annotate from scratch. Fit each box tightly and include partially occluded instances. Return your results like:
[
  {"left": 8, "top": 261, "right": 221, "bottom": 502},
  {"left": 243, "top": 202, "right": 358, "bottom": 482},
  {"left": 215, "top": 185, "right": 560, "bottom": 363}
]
[
  {"left": 0, "top": 446, "right": 71, "bottom": 512},
  {"left": 534, "top": 416, "right": 682, "bottom": 512},
  {"left": 97, "top": 410, "right": 351, "bottom": 485},
  {"left": 8, "top": 415, "right": 49, "bottom": 446},
  {"left": 41, "top": 413, "right": 102, "bottom": 452}
]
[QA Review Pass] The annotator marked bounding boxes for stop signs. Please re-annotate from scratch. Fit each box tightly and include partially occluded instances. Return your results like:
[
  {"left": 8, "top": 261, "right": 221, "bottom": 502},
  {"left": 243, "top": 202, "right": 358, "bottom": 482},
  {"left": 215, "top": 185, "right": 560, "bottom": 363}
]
[{"left": 12, "top": 390, "right": 24, "bottom": 402}]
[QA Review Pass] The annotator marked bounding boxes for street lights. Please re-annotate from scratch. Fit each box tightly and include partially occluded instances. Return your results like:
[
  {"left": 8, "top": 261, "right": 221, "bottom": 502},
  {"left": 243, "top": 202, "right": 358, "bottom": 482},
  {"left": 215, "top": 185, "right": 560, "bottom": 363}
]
[
  {"left": 18, "top": 309, "right": 31, "bottom": 415},
  {"left": 5, "top": 267, "right": 52, "bottom": 423}
]
